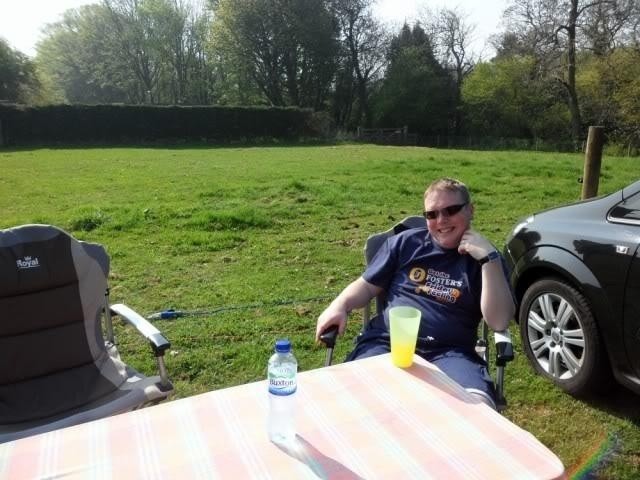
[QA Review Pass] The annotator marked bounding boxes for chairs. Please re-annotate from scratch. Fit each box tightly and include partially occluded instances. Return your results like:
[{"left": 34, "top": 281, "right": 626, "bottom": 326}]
[
  {"left": 320, "top": 214, "right": 514, "bottom": 414},
  {"left": 0, "top": 223, "right": 173, "bottom": 443}
]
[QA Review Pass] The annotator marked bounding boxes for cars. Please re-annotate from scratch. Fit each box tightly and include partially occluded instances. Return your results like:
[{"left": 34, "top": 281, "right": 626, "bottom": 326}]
[{"left": 501, "top": 178, "right": 639, "bottom": 398}]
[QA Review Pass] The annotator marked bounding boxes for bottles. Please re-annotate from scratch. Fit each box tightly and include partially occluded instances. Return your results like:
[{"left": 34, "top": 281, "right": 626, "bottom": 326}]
[{"left": 268, "top": 339, "right": 298, "bottom": 446}]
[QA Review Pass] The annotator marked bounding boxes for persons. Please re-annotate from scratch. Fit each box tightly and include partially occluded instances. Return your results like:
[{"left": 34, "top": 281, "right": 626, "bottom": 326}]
[{"left": 313, "top": 175, "right": 517, "bottom": 411}]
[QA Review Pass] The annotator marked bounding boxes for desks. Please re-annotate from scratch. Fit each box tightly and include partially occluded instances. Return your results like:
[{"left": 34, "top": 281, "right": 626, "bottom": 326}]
[{"left": 0, "top": 352, "right": 565, "bottom": 480}]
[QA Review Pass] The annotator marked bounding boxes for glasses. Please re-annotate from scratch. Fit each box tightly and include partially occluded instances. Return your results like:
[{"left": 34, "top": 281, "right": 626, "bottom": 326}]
[{"left": 422, "top": 202, "right": 465, "bottom": 220}]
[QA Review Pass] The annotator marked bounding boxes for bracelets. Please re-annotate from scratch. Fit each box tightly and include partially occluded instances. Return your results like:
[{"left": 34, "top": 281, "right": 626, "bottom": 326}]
[{"left": 478, "top": 250, "right": 501, "bottom": 266}]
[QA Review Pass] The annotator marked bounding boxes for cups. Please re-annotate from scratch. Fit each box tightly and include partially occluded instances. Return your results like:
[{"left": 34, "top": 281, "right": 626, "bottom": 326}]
[{"left": 389, "top": 305, "right": 421, "bottom": 368}]
